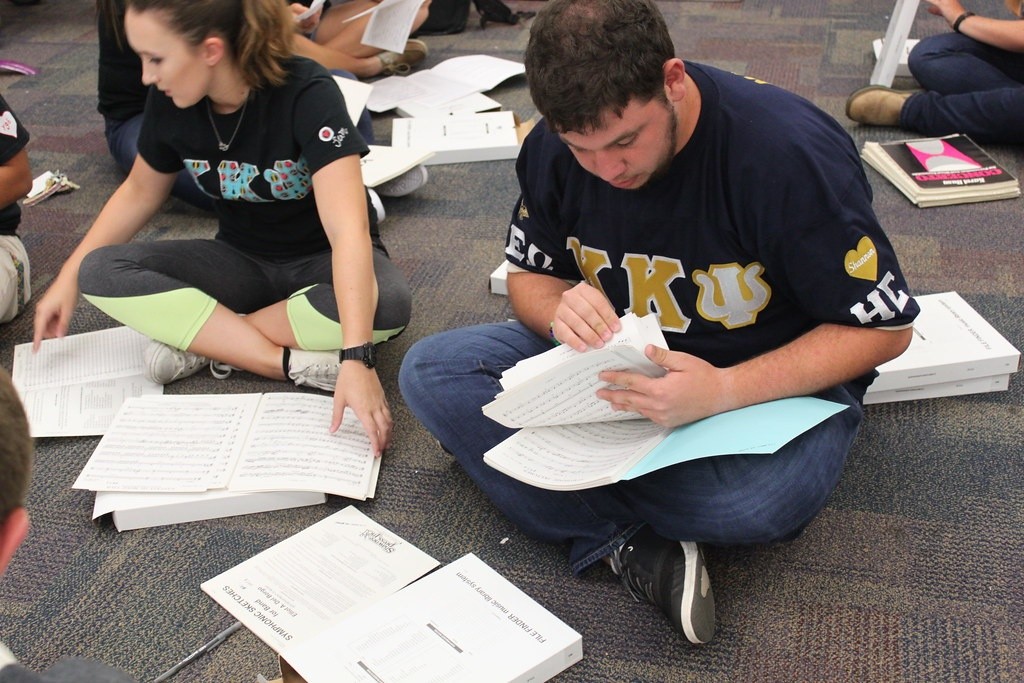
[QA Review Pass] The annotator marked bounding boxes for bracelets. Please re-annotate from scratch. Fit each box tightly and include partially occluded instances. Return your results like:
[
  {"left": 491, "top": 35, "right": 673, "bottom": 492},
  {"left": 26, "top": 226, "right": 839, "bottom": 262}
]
[
  {"left": 954, "top": 12, "right": 975, "bottom": 33},
  {"left": 549, "top": 322, "right": 561, "bottom": 347}
]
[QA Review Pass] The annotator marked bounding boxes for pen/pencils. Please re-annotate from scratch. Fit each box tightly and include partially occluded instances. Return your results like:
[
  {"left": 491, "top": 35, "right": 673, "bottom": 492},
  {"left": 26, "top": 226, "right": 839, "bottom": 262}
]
[{"left": 152, "top": 622, "right": 243, "bottom": 683}]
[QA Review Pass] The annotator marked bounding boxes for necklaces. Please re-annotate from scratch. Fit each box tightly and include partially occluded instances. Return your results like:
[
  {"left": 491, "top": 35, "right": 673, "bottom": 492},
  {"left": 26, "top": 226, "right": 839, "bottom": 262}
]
[{"left": 205, "top": 87, "right": 249, "bottom": 151}]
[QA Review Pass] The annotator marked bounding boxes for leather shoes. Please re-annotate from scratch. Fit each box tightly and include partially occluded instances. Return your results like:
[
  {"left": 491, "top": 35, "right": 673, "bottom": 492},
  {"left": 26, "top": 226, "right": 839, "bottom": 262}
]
[{"left": 845, "top": 83, "right": 922, "bottom": 128}]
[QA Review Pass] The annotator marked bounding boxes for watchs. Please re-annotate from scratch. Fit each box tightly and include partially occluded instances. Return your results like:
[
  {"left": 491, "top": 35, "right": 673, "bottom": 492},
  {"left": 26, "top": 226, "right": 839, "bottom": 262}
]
[{"left": 339, "top": 341, "right": 377, "bottom": 369}]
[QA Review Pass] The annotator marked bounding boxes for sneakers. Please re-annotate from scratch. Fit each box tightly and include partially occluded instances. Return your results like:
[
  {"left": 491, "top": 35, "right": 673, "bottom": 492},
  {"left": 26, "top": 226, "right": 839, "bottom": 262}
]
[
  {"left": 143, "top": 341, "right": 242, "bottom": 384},
  {"left": 287, "top": 349, "right": 341, "bottom": 394},
  {"left": 357, "top": 39, "right": 429, "bottom": 81},
  {"left": 610, "top": 529, "right": 715, "bottom": 644}
]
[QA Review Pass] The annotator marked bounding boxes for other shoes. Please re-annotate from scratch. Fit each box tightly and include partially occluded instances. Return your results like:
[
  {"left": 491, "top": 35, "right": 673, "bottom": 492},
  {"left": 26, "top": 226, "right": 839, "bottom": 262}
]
[
  {"left": 375, "top": 162, "right": 428, "bottom": 197},
  {"left": 367, "top": 189, "right": 387, "bottom": 225}
]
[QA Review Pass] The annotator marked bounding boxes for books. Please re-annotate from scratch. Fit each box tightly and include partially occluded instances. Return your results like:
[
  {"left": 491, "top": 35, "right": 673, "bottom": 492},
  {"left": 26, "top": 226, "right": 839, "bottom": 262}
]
[{"left": 860, "top": 133, "right": 1021, "bottom": 207}]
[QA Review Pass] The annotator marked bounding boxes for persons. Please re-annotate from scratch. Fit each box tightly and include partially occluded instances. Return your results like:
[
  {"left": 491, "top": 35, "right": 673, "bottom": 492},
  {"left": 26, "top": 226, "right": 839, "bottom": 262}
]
[
  {"left": 398, "top": 0, "right": 922, "bottom": 645},
  {"left": 0, "top": 366, "right": 43, "bottom": 683},
  {"left": 94, "top": 0, "right": 433, "bottom": 224},
  {"left": 0, "top": 95, "right": 31, "bottom": 324},
  {"left": 33, "top": 0, "right": 411, "bottom": 458},
  {"left": 845, "top": 0, "right": 1024, "bottom": 140}
]
